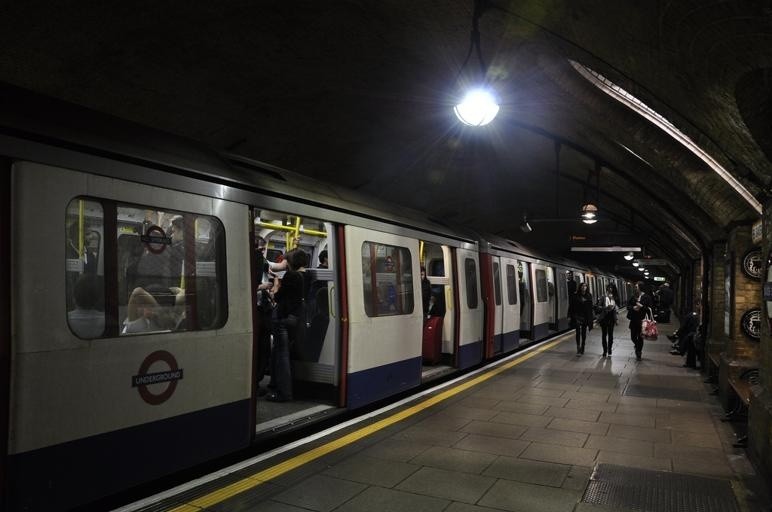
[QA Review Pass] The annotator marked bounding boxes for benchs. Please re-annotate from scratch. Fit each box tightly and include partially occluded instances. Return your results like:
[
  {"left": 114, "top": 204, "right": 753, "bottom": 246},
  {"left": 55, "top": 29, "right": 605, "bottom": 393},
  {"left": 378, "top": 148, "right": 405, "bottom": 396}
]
[{"left": 704, "top": 349, "right": 760, "bottom": 448}]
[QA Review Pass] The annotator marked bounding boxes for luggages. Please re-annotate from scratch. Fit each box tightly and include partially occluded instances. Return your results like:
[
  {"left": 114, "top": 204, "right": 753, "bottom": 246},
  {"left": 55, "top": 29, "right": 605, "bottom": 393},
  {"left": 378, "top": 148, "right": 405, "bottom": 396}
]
[{"left": 653, "top": 309, "right": 668, "bottom": 322}]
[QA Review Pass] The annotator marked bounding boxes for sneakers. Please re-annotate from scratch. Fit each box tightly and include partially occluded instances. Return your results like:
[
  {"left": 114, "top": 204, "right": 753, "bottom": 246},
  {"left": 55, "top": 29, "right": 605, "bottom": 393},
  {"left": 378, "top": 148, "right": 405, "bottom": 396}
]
[{"left": 257, "top": 382, "right": 292, "bottom": 401}]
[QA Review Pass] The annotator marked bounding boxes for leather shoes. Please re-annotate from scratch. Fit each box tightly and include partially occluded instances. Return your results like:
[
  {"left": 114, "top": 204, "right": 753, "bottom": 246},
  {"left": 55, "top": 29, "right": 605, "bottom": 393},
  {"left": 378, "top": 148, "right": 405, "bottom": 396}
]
[
  {"left": 577, "top": 345, "right": 642, "bottom": 361},
  {"left": 666, "top": 331, "right": 684, "bottom": 355}
]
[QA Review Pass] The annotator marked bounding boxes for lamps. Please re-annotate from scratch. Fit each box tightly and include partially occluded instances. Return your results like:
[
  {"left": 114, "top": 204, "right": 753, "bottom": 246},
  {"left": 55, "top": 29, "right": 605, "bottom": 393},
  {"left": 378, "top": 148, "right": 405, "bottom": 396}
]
[
  {"left": 446, "top": 13, "right": 500, "bottom": 129},
  {"left": 574, "top": 168, "right": 604, "bottom": 227}
]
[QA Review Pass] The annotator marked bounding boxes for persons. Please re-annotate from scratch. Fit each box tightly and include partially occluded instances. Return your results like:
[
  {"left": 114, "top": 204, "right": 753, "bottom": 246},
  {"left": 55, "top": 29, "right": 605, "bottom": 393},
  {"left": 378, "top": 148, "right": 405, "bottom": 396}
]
[
  {"left": 420, "top": 265, "right": 433, "bottom": 326},
  {"left": 257, "top": 248, "right": 330, "bottom": 402},
  {"left": 665, "top": 296, "right": 700, "bottom": 369},
  {"left": 591, "top": 282, "right": 620, "bottom": 357},
  {"left": 518, "top": 270, "right": 525, "bottom": 317},
  {"left": 626, "top": 281, "right": 651, "bottom": 361},
  {"left": 567, "top": 271, "right": 576, "bottom": 325},
  {"left": 567, "top": 282, "right": 594, "bottom": 357},
  {"left": 606, "top": 280, "right": 674, "bottom": 323},
  {"left": 67, "top": 217, "right": 215, "bottom": 337},
  {"left": 547, "top": 279, "right": 554, "bottom": 302}
]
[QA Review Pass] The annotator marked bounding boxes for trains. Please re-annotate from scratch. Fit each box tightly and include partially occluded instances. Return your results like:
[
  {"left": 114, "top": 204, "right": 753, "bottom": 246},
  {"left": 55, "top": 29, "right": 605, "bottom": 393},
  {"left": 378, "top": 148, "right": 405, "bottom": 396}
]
[{"left": 0, "top": 73, "right": 640, "bottom": 512}]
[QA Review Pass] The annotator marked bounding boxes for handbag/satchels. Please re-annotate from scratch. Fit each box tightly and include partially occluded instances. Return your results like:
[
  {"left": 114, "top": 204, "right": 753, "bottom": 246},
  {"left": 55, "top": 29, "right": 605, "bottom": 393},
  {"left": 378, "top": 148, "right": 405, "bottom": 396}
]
[
  {"left": 641, "top": 307, "right": 658, "bottom": 341},
  {"left": 259, "top": 289, "right": 281, "bottom": 339},
  {"left": 593, "top": 296, "right": 604, "bottom": 316},
  {"left": 296, "top": 295, "right": 307, "bottom": 341}
]
